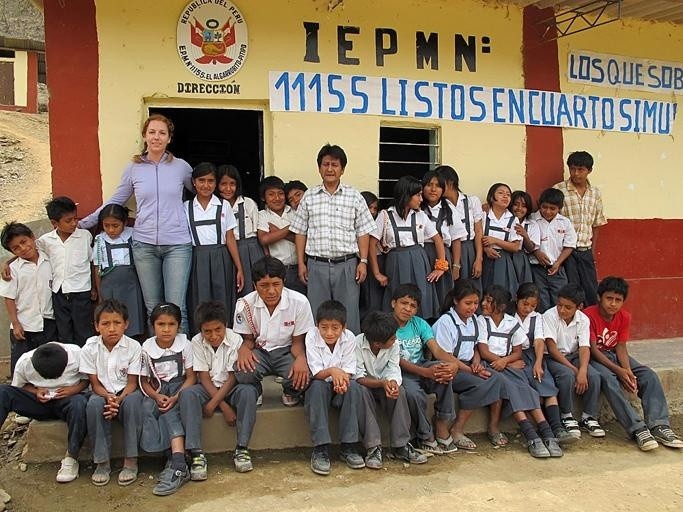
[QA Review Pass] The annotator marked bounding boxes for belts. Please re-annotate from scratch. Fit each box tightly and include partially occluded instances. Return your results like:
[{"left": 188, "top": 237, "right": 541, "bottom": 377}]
[
  {"left": 577, "top": 246, "right": 592, "bottom": 251},
  {"left": 306, "top": 253, "right": 356, "bottom": 263}
]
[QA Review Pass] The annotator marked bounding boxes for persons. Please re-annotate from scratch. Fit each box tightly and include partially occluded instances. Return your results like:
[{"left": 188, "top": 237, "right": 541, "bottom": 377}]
[{"left": 1, "top": 114, "right": 683, "bottom": 496}]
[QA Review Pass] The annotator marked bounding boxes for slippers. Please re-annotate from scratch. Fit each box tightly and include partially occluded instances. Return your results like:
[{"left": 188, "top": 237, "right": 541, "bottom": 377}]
[{"left": 486, "top": 429, "right": 509, "bottom": 447}]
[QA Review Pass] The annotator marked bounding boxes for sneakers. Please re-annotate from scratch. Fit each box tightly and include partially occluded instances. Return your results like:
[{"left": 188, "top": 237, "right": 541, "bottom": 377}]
[
  {"left": 528, "top": 417, "right": 606, "bottom": 458},
  {"left": 56, "top": 454, "right": 208, "bottom": 498},
  {"left": 633, "top": 426, "right": 659, "bottom": 451},
  {"left": 311, "top": 434, "right": 476, "bottom": 475},
  {"left": 282, "top": 393, "right": 299, "bottom": 406},
  {"left": 651, "top": 425, "right": 683, "bottom": 451},
  {"left": 233, "top": 450, "right": 253, "bottom": 472}
]
